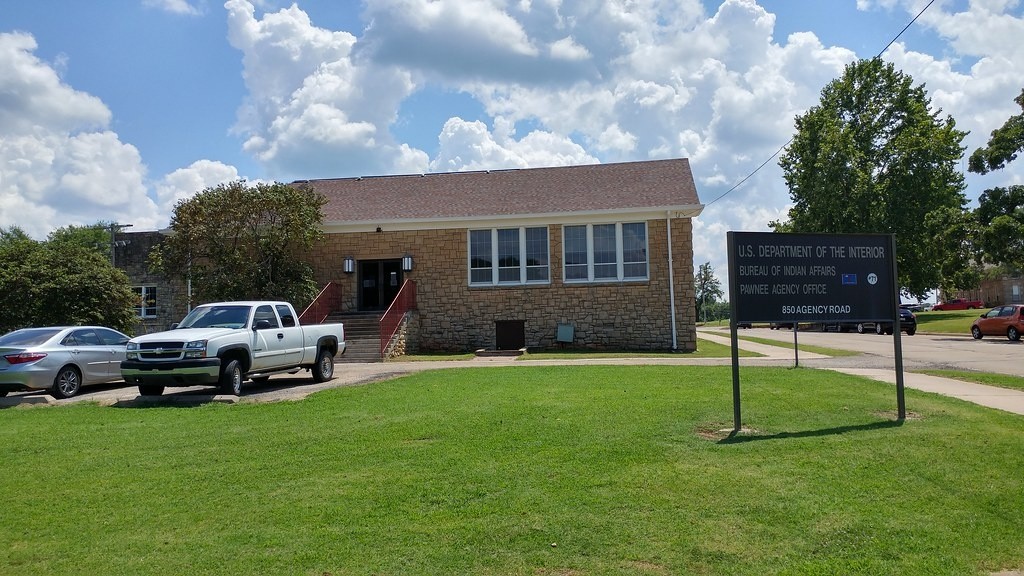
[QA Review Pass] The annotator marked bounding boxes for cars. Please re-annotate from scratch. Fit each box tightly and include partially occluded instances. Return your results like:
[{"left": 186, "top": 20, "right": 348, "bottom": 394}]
[
  {"left": 728, "top": 309, "right": 917, "bottom": 335},
  {"left": 970, "top": 305, "right": 1024, "bottom": 341},
  {"left": 899, "top": 303, "right": 921, "bottom": 312},
  {"left": 0, "top": 326, "right": 139, "bottom": 399}
]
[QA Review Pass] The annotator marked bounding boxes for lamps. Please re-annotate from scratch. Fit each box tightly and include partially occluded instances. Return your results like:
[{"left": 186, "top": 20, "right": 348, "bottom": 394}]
[
  {"left": 402, "top": 253, "right": 412, "bottom": 273},
  {"left": 343, "top": 256, "right": 355, "bottom": 274}
]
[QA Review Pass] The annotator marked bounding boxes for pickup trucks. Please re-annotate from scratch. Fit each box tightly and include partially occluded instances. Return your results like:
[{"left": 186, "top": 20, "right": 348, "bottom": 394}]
[
  {"left": 120, "top": 301, "right": 345, "bottom": 396},
  {"left": 932, "top": 298, "right": 984, "bottom": 310}
]
